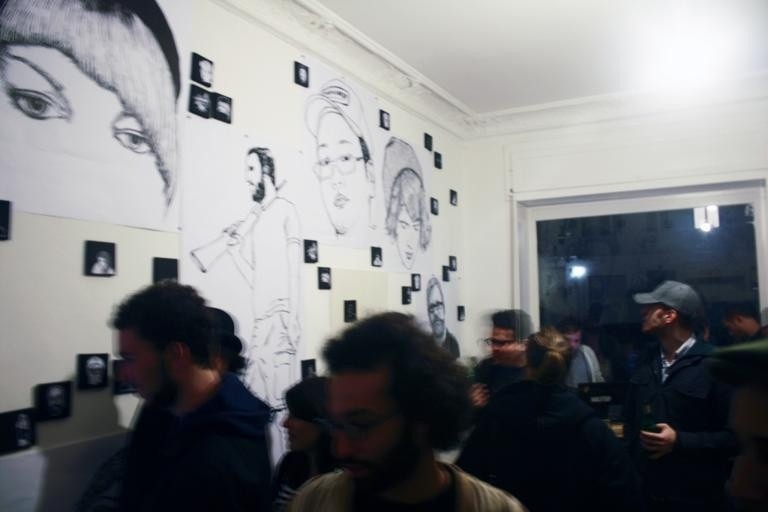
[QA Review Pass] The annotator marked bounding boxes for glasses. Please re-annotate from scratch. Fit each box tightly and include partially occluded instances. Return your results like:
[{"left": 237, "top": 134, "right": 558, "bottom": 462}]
[
  {"left": 484, "top": 338, "right": 516, "bottom": 346},
  {"left": 318, "top": 411, "right": 405, "bottom": 435}
]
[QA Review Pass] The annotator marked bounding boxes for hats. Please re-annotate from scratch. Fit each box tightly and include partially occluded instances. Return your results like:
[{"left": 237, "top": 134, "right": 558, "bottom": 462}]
[{"left": 633, "top": 281, "right": 703, "bottom": 317}]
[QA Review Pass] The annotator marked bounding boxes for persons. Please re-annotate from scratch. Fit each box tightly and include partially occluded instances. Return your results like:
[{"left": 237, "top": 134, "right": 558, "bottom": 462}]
[
  {"left": 426, "top": 278, "right": 459, "bottom": 360},
  {"left": 86, "top": 356, "right": 106, "bottom": 386},
  {"left": 557, "top": 315, "right": 605, "bottom": 395},
  {"left": 263, "top": 375, "right": 338, "bottom": 512},
  {"left": 305, "top": 79, "right": 377, "bottom": 244},
  {"left": 703, "top": 301, "right": 762, "bottom": 348},
  {"left": 45, "top": 386, "right": 63, "bottom": 413},
  {"left": 107, "top": 279, "right": 271, "bottom": 510},
  {"left": 576, "top": 303, "right": 662, "bottom": 382},
  {"left": 206, "top": 306, "right": 271, "bottom": 462},
  {"left": 382, "top": 137, "right": 432, "bottom": 269},
  {"left": 453, "top": 330, "right": 644, "bottom": 512},
  {"left": 286, "top": 312, "right": 528, "bottom": 512},
  {"left": 224, "top": 148, "right": 302, "bottom": 466},
  {"left": 0, "top": 0, "right": 179, "bottom": 232},
  {"left": 91, "top": 251, "right": 113, "bottom": 274},
  {"left": 625, "top": 280, "right": 744, "bottom": 511},
  {"left": 461, "top": 310, "right": 529, "bottom": 425}
]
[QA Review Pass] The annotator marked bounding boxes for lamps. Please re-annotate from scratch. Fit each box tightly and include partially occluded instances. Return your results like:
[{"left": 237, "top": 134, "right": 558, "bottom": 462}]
[{"left": 691, "top": 204, "right": 720, "bottom": 231}]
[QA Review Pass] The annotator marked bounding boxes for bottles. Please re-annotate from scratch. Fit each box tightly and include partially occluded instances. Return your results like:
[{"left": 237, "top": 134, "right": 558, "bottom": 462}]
[{"left": 639, "top": 399, "right": 657, "bottom": 435}]
